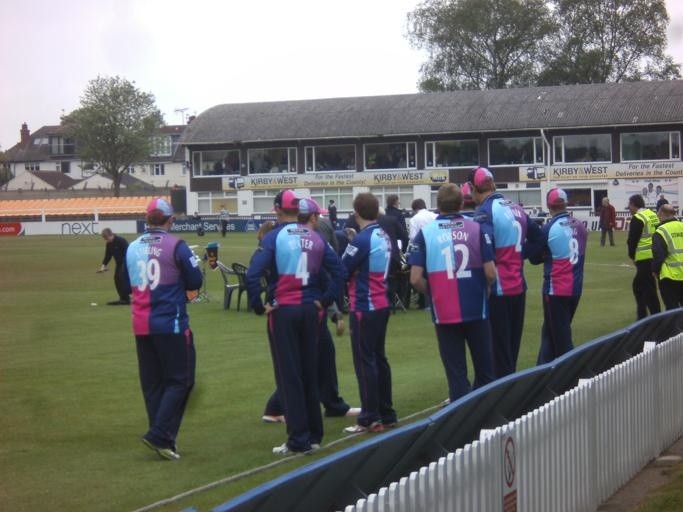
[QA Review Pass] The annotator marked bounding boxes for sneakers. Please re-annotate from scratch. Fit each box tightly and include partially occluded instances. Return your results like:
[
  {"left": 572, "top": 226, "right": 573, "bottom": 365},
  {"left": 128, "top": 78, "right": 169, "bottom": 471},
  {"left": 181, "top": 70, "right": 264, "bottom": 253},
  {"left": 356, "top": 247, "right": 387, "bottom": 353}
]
[
  {"left": 346, "top": 407, "right": 361, "bottom": 416},
  {"left": 336, "top": 311, "right": 345, "bottom": 336},
  {"left": 345, "top": 419, "right": 396, "bottom": 432},
  {"left": 262, "top": 415, "right": 286, "bottom": 423},
  {"left": 273, "top": 443, "right": 319, "bottom": 454},
  {"left": 143, "top": 435, "right": 180, "bottom": 461}
]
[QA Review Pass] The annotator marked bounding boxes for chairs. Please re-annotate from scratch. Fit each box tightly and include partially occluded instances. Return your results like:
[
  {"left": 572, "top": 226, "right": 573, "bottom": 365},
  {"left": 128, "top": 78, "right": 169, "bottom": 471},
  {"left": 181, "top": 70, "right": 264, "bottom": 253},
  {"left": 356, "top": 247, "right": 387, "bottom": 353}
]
[{"left": 216, "top": 261, "right": 268, "bottom": 313}]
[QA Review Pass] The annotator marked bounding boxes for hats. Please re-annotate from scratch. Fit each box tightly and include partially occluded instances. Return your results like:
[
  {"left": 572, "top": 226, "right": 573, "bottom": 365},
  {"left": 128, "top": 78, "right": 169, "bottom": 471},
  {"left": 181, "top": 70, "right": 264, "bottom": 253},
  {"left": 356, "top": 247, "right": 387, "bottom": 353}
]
[
  {"left": 147, "top": 199, "right": 172, "bottom": 216},
  {"left": 547, "top": 188, "right": 568, "bottom": 206},
  {"left": 298, "top": 198, "right": 328, "bottom": 214},
  {"left": 472, "top": 166, "right": 494, "bottom": 186},
  {"left": 462, "top": 182, "right": 475, "bottom": 202},
  {"left": 272, "top": 191, "right": 299, "bottom": 211}
]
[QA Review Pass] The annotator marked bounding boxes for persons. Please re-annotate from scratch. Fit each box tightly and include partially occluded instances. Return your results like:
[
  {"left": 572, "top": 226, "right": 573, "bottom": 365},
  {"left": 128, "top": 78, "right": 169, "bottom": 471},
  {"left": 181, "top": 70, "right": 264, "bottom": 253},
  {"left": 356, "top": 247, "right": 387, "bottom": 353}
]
[
  {"left": 260, "top": 195, "right": 551, "bottom": 312},
  {"left": 219, "top": 205, "right": 230, "bottom": 238},
  {"left": 342, "top": 192, "right": 401, "bottom": 437},
  {"left": 599, "top": 196, "right": 683, "bottom": 324},
  {"left": 260, "top": 197, "right": 363, "bottom": 423},
  {"left": 407, "top": 182, "right": 498, "bottom": 409},
  {"left": 192, "top": 210, "right": 201, "bottom": 219},
  {"left": 467, "top": 165, "right": 547, "bottom": 380},
  {"left": 95, "top": 227, "right": 132, "bottom": 306},
  {"left": 536, "top": 186, "right": 589, "bottom": 366},
  {"left": 640, "top": 182, "right": 664, "bottom": 208},
  {"left": 125, "top": 198, "right": 203, "bottom": 462},
  {"left": 245, "top": 189, "right": 349, "bottom": 459},
  {"left": 198, "top": 140, "right": 637, "bottom": 175}
]
